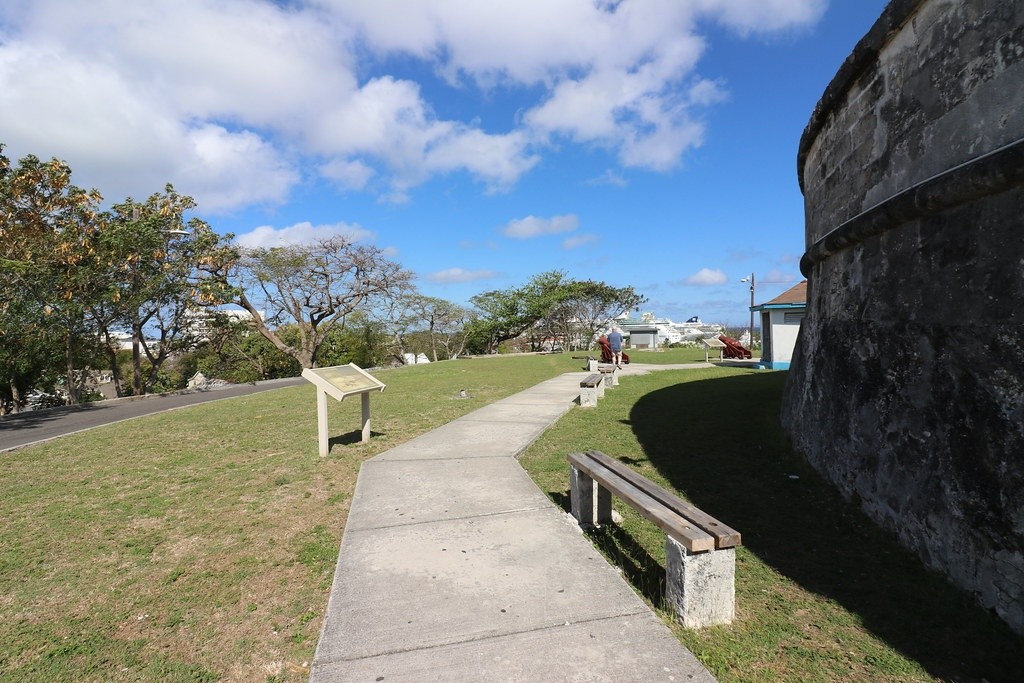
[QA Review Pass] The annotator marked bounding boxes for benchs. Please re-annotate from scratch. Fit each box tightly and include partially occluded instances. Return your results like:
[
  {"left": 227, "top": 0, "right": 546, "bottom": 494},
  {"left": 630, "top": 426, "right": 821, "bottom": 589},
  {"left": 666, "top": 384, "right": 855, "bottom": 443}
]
[
  {"left": 600, "top": 364, "right": 619, "bottom": 389},
  {"left": 566, "top": 450, "right": 742, "bottom": 630},
  {"left": 580, "top": 373, "right": 606, "bottom": 407}
]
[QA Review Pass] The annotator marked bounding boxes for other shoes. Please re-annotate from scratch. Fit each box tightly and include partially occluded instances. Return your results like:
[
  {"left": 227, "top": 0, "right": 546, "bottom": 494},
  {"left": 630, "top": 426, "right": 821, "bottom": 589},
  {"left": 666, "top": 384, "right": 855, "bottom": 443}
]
[{"left": 616, "top": 364, "right": 622, "bottom": 370}]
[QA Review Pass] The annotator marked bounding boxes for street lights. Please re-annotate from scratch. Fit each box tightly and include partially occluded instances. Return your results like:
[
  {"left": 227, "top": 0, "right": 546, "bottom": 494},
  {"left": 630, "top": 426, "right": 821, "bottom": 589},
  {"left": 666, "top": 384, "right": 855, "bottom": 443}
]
[
  {"left": 741, "top": 273, "right": 754, "bottom": 350},
  {"left": 133, "top": 230, "right": 191, "bottom": 396}
]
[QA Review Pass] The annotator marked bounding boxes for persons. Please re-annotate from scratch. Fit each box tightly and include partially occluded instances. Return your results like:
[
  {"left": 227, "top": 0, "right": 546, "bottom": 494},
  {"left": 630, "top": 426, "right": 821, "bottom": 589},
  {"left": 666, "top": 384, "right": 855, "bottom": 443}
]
[{"left": 606, "top": 325, "right": 624, "bottom": 370}]
